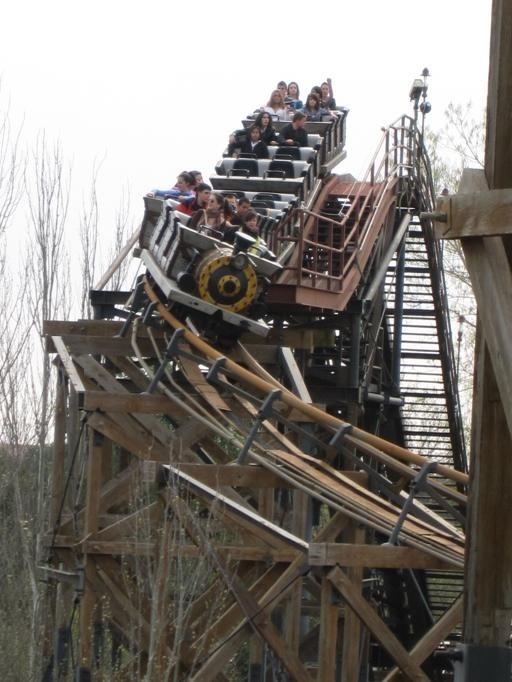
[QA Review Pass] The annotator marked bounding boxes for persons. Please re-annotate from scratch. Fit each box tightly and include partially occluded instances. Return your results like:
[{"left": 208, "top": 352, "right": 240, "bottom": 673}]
[{"left": 148, "top": 76, "right": 336, "bottom": 256}]
